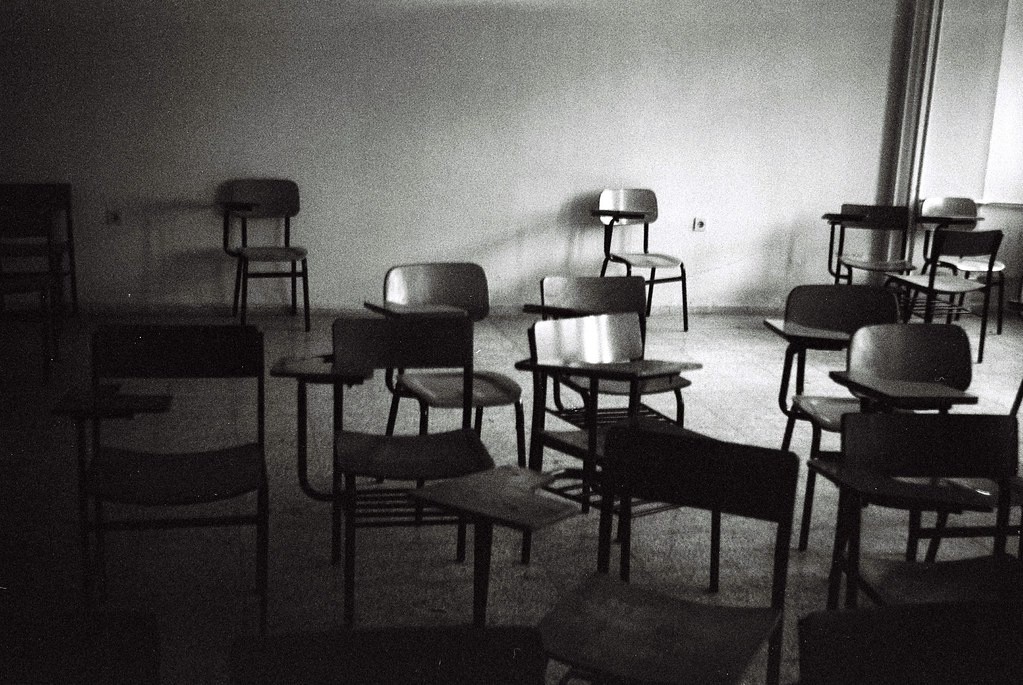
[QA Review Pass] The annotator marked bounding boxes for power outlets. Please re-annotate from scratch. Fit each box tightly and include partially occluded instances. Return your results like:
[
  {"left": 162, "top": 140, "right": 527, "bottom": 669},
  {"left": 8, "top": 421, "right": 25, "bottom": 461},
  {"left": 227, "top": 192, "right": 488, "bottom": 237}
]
[
  {"left": 106, "top": 209, "right": 125, "bottom": 226},
  {"left": 693, "top": 216, "right": 707, "bottom": 232}
]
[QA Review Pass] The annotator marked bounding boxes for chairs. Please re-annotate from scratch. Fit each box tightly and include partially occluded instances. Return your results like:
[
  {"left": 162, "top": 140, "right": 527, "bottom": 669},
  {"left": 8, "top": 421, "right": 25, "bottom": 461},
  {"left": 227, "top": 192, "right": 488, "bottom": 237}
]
[{"left": 0, "top": 164, "right": 1021, "bottom": 684}]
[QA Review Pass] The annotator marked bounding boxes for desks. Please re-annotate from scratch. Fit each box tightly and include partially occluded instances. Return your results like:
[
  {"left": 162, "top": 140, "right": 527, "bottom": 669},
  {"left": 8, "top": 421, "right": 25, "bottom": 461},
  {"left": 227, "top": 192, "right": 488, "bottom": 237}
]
[
  {"left": 52, "top": 384, "right": 175, "bottom": 486},
  {"left": 364, "top": 299, "right": 466, "bottom": 388},
  {"left": 809, "top": 459, "right": 994, "bottom": 610},
  {"left": 758, "top": 315, "right": 861, "bottom": 452},
  {"left": 220, "top": 201, "right": 261, "bottom": 252},
  {"left": 272, "top": 351, "right": 370, "bottom": 503},
  {"left": 822, "top": 374, "right": 983, "bottom": 557},
  {"left": 554, "top": 365, "right": 703, "bottom": 511},
  {"left": 591, "top": 208, "right": 645, "bottom": 256},
  {"left": 821, "top": 213, "right": 872, "bottom": 269},
  {"left": 411, "top": 466, "right": 597, "bottom": 626},
  {"left": 520, "top": 304, "right": 595, "bottom": 406},
  {"left": 919, "top": 211, "right": 985, "bottom": 261}
]
